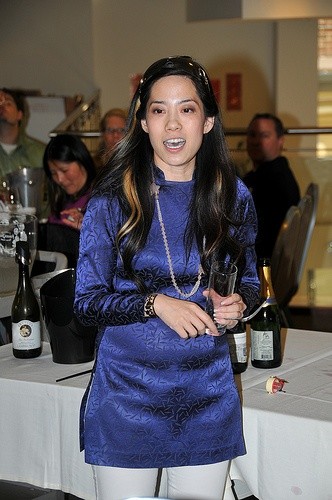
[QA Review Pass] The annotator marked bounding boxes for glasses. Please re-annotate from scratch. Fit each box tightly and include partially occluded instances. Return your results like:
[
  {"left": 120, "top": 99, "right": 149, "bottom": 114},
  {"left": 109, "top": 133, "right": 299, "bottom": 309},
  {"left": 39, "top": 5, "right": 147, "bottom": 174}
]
[
  {"left": 141, "top": 58, "right": 211, "bottom": 92},
  {"left": 104, "top": 127, "right": 126, "bottom": 134}
]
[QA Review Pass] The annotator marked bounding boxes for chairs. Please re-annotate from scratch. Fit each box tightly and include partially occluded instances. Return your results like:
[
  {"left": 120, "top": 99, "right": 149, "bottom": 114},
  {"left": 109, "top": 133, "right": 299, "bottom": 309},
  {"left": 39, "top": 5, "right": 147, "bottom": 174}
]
[{"left": 270, "top": 182, "right": 319, "bottom": 311}]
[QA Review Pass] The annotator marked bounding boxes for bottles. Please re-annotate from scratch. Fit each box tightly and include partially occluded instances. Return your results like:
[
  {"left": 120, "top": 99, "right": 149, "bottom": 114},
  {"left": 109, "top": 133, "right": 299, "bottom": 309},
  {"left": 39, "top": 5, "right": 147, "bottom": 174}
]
[
  {"left": 250, "top": 258, "right": 282, "bottom": 369},
  {"left": 225, "top": 319, "right": 248, "bottom": 375},
  {"left": 11, "top": 241, "right": 42, "bottom": 359}
]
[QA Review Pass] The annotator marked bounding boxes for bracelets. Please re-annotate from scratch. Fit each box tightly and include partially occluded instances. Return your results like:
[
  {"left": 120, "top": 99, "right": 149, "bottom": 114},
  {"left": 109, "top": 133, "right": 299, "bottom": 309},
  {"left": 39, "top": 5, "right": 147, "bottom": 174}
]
[{"left": 144, "top": 293, "right": 158, "bottom": 316}]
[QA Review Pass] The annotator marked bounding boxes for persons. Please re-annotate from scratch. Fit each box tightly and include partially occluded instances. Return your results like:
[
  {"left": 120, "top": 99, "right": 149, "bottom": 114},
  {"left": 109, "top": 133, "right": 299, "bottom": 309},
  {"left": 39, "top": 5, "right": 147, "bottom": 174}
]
[
  {"left": 40, "top": 135, "right": 100, "bottom": 323},
  {"left": 74, "top": 55, "right": 261, "bottom": 500},
  {"left": 0, "top": 87, "right": 48, "bottom": 205},
  {"left": 97, "top": 108, "right": 128, "bottom": 167},
  {"left": 241, "top": 113, "right": 300, "bottom": 280}
]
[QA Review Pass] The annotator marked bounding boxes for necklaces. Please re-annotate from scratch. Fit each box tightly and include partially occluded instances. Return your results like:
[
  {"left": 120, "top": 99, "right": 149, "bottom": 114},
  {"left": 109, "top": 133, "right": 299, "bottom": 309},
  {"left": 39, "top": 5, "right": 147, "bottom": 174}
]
[{"left": 151, "top": 163, "right": 204, "bottom": 298}]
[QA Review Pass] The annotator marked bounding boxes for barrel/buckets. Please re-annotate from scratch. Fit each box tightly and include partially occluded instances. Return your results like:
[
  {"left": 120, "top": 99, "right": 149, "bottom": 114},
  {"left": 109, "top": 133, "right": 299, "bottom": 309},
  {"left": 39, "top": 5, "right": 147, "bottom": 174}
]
[{"left": 31, "top": 269, "right": 98, "bottom": 365}]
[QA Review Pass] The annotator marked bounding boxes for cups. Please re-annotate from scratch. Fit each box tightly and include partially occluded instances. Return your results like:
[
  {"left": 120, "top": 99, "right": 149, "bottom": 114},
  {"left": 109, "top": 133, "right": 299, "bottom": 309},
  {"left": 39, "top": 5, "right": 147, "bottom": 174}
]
[
  {"left": 7, "top": 167, "right": 45, "bottom": 217},
  {"left": 205, "top": 261, "right": 238, "bottom": 336},
  {"left": 0, "top": 211, "right": 38, "bottom": 296}
]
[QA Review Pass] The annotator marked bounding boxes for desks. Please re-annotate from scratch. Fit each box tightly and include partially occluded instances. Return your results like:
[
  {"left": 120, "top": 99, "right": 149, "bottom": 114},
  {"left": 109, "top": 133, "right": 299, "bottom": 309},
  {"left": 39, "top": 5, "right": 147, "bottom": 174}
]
[{"left": 0, "top": 328, "right": 332, "bottom": 499}]
